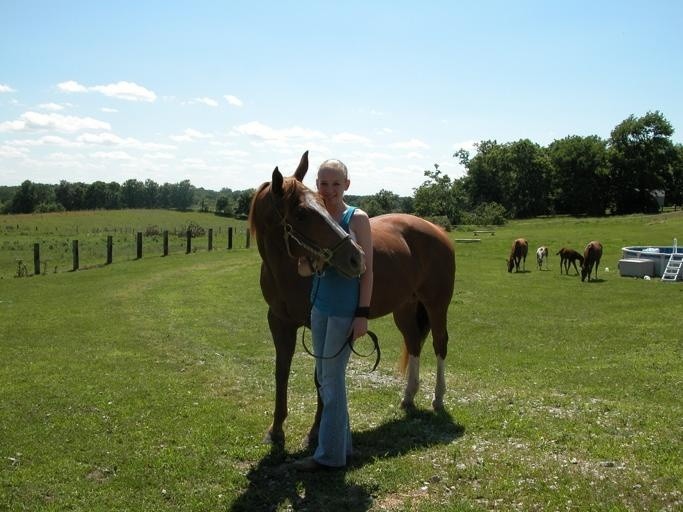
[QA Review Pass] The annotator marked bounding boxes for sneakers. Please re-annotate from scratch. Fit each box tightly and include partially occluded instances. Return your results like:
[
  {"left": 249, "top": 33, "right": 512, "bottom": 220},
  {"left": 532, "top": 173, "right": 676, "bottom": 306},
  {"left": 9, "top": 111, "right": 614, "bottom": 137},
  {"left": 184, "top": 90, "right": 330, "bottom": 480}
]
[{"left": 292, "top": 456, "right": 343, "bottom": 472}]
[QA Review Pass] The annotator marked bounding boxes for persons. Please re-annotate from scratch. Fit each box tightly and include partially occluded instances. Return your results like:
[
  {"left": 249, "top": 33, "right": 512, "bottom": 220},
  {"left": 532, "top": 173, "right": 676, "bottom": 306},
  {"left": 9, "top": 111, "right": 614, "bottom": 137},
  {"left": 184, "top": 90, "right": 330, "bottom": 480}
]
[{"left": 286, "top": 157, "right": 376, "bottom": 481}]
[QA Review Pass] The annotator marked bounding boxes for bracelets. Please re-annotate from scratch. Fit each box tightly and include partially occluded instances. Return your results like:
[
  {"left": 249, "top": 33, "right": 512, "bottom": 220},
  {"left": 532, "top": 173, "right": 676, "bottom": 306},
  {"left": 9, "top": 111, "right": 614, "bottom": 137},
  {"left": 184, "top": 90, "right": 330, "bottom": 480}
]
[{"left": 356, "top": 304, "right": 372, "bottom": 319}]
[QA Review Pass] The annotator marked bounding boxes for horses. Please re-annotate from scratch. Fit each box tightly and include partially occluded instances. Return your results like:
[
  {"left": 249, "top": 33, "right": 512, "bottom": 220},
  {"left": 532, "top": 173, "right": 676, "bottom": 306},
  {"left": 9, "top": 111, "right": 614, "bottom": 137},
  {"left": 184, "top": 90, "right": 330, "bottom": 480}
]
[
  {"left": 556, "top": 247, "right": 584, "bottom": 276},
  {"left": 536, "top": 246, "right": 548, "bottom": 271},
  {"left": 247, "top": 149, "right": 456, "bottom": 453},
  {"left": 505, "top": 238, "right": 528, "bottom": 273},
  {"left": 580, "top": 240, "right": 602, "bottom": 282}
]
